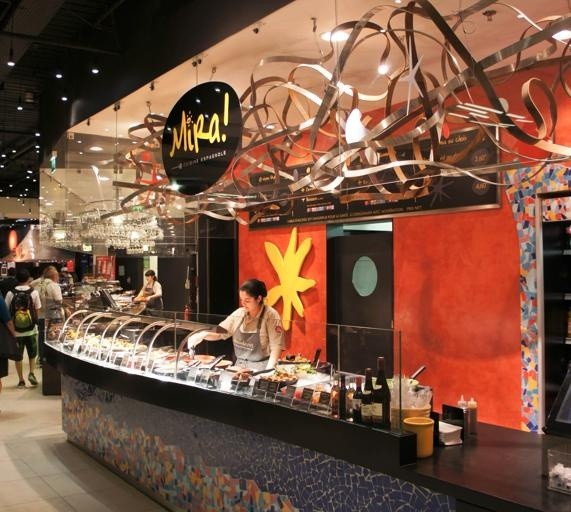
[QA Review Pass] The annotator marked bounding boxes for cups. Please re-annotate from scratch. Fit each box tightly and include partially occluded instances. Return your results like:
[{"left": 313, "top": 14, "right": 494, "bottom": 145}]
[{"left": 403, "top": 417, "right": 435, "bottom": 459}]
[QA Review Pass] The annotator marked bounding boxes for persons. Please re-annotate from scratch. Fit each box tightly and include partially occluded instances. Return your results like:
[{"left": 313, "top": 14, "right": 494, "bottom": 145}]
[
  {"left": 0, "top": 266, "right": 73, "bottom": 394},
  {"left": 187, "top": 278, "right": 287, "bottom": 371},
  {"left": 134, "top": 269, "right": 164, "bottom": 309}
]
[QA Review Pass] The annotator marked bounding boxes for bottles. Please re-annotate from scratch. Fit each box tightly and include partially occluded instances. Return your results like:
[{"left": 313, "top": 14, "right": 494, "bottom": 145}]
[
  {"left": 468, "top": 395, "right": 477, "bottom": 433},
  {"left": 458, "top": 394, "right": 468, "bottom": 437},
  {"left": 330, "top": 374, "right": 338, "bottom": 415},
  {"left": 361, "top": 368, "right": 375, "bottom": 420},
  {"left": 374, "top": 356, "right": 391, "bottom": 428},
  {"left": 352, "top": 376, "right": 363, "bottom": 423},
  {"left": 337, "top": 374, "right": 347, "bottom": 418}
]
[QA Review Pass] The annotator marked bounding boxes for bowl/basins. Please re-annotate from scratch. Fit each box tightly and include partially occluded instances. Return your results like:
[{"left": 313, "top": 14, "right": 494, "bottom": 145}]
[{"left": 391, "top": 404, "right": 431, "bottom": 429}]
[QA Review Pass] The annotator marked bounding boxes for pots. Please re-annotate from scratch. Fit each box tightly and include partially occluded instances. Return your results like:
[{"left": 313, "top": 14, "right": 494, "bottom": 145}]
[{"left": 361, "top": 365, "right": 426, "bottom": 394}]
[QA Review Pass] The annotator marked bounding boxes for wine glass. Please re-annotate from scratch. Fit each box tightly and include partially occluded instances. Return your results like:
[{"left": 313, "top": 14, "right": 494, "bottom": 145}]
[{"left": 67, "top": 207, "right": 164, "bottom": 255}]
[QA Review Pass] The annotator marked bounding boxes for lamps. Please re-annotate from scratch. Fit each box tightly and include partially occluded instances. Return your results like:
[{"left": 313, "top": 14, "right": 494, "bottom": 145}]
[{"left": 22, "top": 197, "right": 32, "bottom": 216}]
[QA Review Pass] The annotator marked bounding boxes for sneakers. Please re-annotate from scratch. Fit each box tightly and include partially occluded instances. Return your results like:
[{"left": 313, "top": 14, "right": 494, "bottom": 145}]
[
  {"left": 28, "top": 373, "right": 38, "bottom": 385},
  {"left": 17, "top": 381, "right": 25, "bottom": 389}
]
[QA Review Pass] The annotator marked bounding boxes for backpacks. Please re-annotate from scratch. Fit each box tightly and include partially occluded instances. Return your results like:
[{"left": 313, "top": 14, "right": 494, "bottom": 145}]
[{"left": 10, "top": 287, "right": 36, "bottom": 332}]
